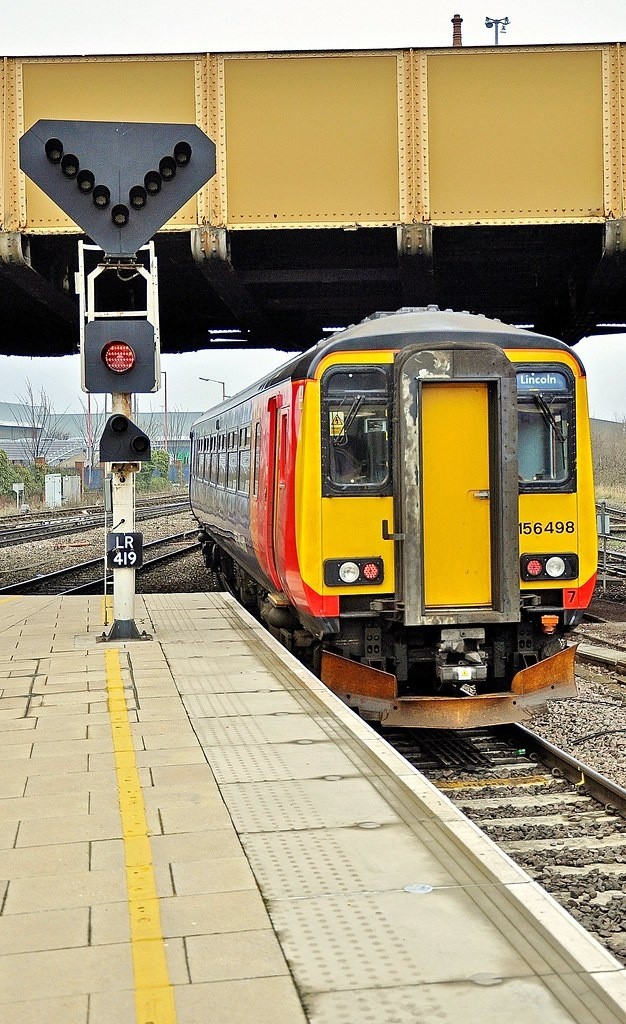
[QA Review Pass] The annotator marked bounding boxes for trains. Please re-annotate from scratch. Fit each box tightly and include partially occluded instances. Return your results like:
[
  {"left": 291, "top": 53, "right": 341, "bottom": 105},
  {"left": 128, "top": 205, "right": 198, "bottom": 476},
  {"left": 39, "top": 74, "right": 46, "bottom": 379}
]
[{"left": 191, "top": 309, "right": 596, "bottom": 732}]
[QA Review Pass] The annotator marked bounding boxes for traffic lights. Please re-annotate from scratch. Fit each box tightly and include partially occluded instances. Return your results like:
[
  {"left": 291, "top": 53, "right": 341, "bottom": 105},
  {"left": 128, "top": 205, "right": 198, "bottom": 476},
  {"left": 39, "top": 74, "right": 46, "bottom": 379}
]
[{"left": 83, "top": 319, "right": 157, "bottom": 393}]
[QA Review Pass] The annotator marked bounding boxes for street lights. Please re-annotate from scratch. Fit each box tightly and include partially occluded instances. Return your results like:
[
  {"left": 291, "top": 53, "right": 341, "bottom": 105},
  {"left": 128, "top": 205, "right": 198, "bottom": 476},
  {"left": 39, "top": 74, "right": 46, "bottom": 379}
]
[
  {"left": 485, "top": 17, "right": 510, "bottom": 44},
  {"left": 198, "top": 376, "right": 225, "bottom": 400}
]
